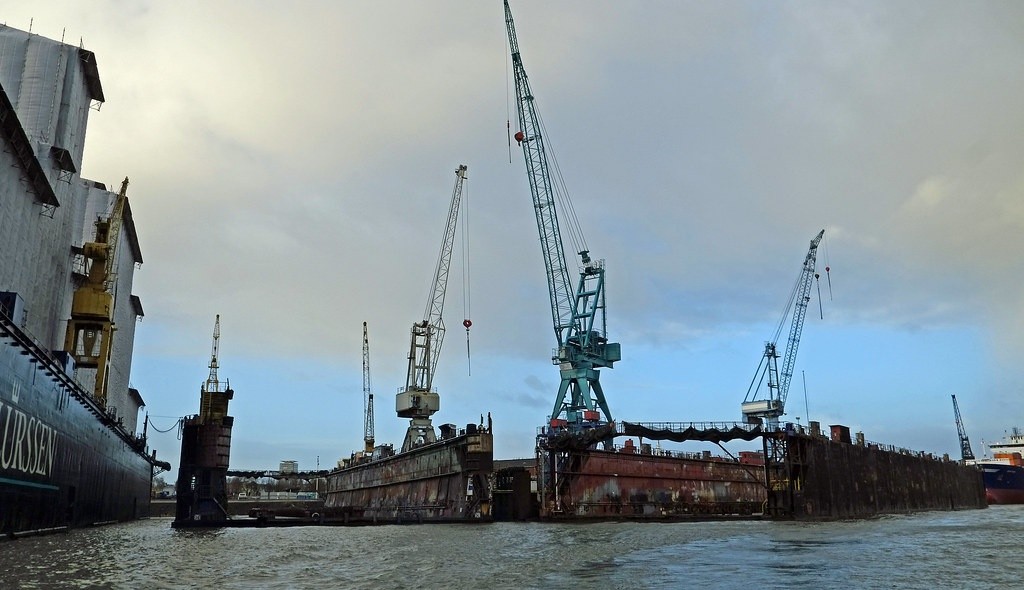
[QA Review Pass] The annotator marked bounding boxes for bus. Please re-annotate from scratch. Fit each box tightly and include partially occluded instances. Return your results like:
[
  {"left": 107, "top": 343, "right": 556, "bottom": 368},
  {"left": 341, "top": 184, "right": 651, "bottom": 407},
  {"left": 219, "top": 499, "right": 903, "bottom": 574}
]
[{"left": 297, "top": 492, "right": 320, "bottom": 500}]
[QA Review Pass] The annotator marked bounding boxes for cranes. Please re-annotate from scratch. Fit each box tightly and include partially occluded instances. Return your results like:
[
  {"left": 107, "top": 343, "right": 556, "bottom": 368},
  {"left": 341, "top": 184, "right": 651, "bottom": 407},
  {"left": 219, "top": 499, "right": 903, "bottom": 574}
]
[
  {"left": 395, "top": 162, "right": 468, "bottom": 453},
  {"left": 362, "top": 321, "right": 376, "bottom": 453},
  {"left": 498, "top": 2, "right": 624, "bottom": 451},
  {"left": 950, "top": 393, "right": 976, "bottom": 467},
  {"left": 739, "top": 227, "right": 828, "bottom": 432}
]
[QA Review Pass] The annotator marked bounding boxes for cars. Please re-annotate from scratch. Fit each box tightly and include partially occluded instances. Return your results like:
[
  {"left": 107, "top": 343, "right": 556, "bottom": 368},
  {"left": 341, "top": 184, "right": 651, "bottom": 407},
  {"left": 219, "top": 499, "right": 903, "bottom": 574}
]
[{"left": 237, "top": 492, "right": 247, "bottom": 500}]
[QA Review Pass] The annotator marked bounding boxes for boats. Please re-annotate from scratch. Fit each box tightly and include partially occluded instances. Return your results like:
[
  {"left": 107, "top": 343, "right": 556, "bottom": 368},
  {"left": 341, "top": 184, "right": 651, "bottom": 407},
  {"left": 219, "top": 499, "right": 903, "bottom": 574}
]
[{"left": 975, "top": 425, "right": 1024, "bottom": 506}]
